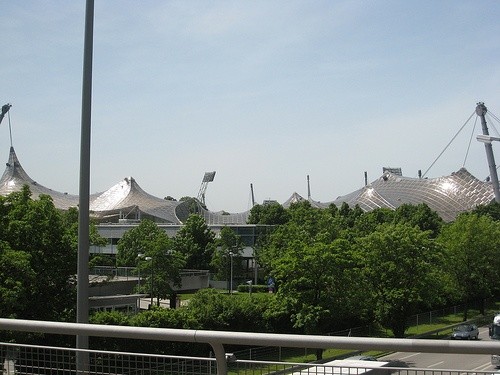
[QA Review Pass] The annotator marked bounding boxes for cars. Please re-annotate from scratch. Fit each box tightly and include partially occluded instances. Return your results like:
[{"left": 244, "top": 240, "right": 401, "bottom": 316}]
[
  {"left": 345, "top": 355, "right": 378, "bottom": 362},
  {"left": 450, "top": 323, "right": 480, "bottom": 340}
]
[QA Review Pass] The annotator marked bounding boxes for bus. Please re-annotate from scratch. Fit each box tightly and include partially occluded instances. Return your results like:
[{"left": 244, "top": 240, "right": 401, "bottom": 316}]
[{"left": 489, "top": 314, "right": 500, "bottom": 368}]
